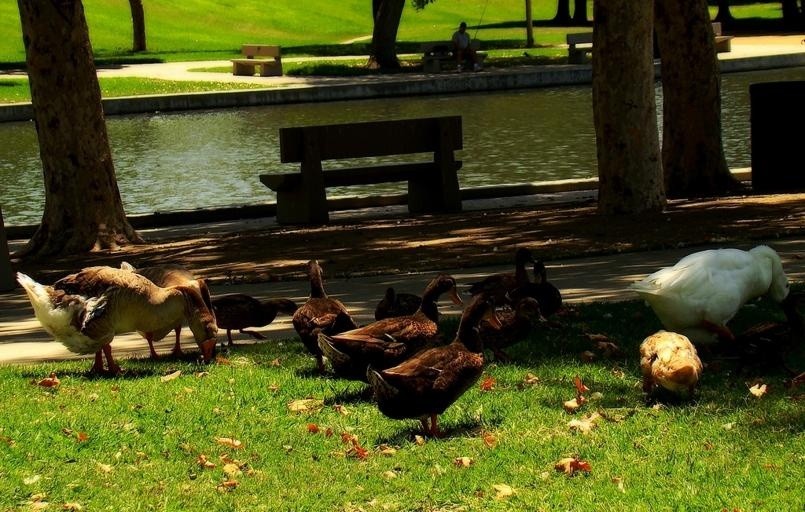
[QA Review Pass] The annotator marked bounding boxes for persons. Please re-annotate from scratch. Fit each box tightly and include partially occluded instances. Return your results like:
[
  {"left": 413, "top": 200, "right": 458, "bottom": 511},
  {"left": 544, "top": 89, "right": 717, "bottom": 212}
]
[{"left": 452, "top": 22, "right": 483, "bottom": 73}]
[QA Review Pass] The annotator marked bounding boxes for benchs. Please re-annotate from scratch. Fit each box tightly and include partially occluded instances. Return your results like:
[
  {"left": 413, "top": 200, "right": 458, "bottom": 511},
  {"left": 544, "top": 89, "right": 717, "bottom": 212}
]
[
  {"left": 566, "top": 32, "right": 593, "bottom": 63},
  {"left": 712, "top": 22, "right": 733, "bottom": 54},
  {"left": 230, "top": 44, "right": 282, "bottom": 78},
  {"left": 422, "top": 39, "right": 488, "bottom": 74},
  {"left": 260, "top": 114, "right": 465, "bottom": 219}
]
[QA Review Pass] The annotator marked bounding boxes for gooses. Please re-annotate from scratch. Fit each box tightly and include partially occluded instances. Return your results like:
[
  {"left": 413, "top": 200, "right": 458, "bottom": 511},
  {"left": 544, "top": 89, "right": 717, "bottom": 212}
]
[{"left": 624, "top": 243, "right": 793, "bottom": 339}]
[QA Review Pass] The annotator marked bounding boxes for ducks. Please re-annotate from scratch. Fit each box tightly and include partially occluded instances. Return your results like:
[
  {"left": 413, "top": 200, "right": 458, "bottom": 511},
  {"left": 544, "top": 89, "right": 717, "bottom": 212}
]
[
  {"left": 12, "top": 261, "right": 294, "bottom": 379},
  {"left": 634, "top": 325, "right": 708, "bottom": 402},
  {"left": 296, "top": 258, "right": 562, "bottom": 446}
]
